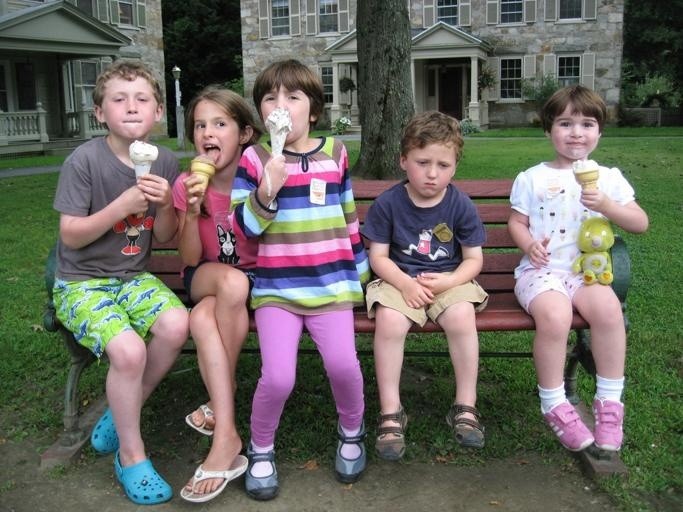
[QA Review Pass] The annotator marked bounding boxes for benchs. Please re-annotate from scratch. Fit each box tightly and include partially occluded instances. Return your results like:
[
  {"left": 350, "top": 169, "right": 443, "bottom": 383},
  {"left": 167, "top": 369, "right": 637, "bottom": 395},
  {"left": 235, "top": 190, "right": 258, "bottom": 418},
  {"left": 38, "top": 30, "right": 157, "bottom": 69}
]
[{"left": 37, "top": 173, "right": 631, "bottom": 485}]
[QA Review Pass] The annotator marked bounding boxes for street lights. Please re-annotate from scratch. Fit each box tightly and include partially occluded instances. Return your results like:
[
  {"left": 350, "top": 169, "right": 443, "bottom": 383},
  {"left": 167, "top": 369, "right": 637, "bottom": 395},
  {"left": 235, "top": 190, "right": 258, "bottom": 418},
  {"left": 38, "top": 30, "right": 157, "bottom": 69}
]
[{"left": 171, "top": 64, "right": 189, "bottom": 148}]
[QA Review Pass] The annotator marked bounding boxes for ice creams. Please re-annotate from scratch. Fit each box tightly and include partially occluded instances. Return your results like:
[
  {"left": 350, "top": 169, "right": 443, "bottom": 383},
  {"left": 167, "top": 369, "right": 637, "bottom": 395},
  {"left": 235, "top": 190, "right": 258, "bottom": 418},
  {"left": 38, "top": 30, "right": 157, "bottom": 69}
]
[
  {"left": 264, "top": 110, "right": 293, "bottom": 156},
  {"left": 573, "top": 158, "right": 601, "bottom": 193},
  {"left": 129, "top": 141, "right": 158, "bottom": 182},
  {"left": 190, "top": 155, "right": 215, "bottom": 198}
]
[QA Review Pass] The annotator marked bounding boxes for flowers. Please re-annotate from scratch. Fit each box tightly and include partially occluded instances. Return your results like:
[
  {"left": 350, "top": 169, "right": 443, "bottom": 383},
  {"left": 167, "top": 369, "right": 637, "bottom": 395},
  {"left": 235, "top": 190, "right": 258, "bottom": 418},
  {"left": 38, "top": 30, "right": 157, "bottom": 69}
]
[{"left": 329, "top": 117, "right": 351, "bottom": 131}]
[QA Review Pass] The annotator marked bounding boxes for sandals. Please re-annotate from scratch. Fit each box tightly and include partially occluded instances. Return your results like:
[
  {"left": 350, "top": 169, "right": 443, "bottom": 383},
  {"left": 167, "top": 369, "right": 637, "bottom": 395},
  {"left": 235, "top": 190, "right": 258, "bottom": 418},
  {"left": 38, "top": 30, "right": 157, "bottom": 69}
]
[
  {"left": 91, "top": 407, "right": 120, "bottom": 453},
  {"left": 114, "top": 448, "right": 173, "bottom": 505}
]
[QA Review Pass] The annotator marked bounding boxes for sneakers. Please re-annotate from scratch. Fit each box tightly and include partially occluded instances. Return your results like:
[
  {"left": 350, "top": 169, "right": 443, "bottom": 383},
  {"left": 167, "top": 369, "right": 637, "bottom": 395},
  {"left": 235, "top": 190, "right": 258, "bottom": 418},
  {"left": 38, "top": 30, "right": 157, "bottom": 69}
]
[
  {"left": 541, "top": 398, "right": 595, "bottom": 452},
  {"left": 375, "top": 403, "right": 408, "bottom": 461},
  {"left": 245, "top": 442, "right": 279, "bottom": 501},
  {"left": 445, "top": 404, "right": 485, "bottom": 448},
  {"left": 334, "top": 417, "right": 366, "bottom": 484},
  {"left": 592, "top": 393, "right": 625, "bottom": 451}
]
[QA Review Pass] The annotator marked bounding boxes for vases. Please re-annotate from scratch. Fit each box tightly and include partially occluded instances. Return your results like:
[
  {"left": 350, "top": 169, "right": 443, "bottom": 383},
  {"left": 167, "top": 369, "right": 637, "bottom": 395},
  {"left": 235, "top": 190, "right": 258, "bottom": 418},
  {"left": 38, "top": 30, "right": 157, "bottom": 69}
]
[{"left": 336, "top": 130, "right": 345, "bottom": 135}]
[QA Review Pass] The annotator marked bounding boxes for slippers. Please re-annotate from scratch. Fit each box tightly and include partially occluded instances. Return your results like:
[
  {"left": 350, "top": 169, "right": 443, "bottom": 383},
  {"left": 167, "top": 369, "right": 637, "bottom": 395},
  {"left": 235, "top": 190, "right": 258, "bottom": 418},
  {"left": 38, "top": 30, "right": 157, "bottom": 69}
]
[
  {"left": 180, "top": 454, "right": 248, "bottom": 503},
  {"left": 185, "top": 404, "right": 215, "bottom": 436}
]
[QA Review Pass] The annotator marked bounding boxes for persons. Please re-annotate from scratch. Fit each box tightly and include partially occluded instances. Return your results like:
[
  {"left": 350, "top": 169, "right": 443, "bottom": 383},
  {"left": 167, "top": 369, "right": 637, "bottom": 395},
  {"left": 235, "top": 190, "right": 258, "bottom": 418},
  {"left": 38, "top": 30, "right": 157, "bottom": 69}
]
[
  {"left": 52, "top": 62, "right": 189, "bottom": 506},
  {"left": 359, "top": 110, "right": 490, "bottom": 460},
  {"left": 171, "top": 88, "right": 258, "bottom": 503},
  {"left": 507, "top": 84, "right": 649, "bottom": 452},
  {"left": 225, "top": 60, "right": 371, "bottom": 500}
]
